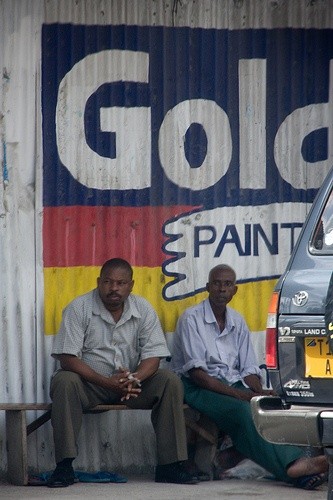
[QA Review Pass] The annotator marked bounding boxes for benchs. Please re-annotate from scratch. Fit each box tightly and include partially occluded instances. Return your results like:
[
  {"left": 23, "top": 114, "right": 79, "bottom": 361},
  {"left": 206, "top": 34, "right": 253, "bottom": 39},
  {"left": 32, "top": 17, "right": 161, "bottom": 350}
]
[{"left": 0, "top": 402, "right": 218, "bottom": 486}]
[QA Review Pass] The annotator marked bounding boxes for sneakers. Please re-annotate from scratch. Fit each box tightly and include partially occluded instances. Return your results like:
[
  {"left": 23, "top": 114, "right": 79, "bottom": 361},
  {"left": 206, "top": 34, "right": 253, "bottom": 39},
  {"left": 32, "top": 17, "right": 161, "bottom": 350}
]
[{"left": 187, "top": 464, "right": 210, "bottom": 480}]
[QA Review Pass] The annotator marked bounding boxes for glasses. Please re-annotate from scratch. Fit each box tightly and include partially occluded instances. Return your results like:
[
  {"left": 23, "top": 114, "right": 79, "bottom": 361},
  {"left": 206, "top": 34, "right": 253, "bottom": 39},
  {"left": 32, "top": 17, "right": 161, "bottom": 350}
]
[
  {"left": 209, "top": 281, "right": 235, "bottom": 287},
  {"left": 100, "top": 278, "right": 131, "bottom": 287}
]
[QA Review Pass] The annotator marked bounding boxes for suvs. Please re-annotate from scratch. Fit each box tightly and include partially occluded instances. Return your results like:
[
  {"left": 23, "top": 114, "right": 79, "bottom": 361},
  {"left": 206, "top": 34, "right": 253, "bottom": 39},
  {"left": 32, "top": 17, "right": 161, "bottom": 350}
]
[{"left": 249, "top": 165, "right": 333, "bottom": 461}]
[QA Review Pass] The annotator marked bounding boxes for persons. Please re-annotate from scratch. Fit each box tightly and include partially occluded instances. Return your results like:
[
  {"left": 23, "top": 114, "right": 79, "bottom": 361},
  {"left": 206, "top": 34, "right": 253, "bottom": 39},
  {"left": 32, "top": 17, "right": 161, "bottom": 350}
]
[
  {"left": 171, "top": 264, "right": 330, "bottom": 490},
  {"left": 47, "top": 258, "right": 209, "bottom": 487}
]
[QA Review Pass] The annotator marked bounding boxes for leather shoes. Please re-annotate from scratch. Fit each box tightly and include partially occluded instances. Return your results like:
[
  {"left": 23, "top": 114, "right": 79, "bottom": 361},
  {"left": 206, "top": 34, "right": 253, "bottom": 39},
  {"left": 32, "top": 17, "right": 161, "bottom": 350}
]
[
  {"left": 154, "top": 464, "right": 198, "bottom": 484},
  {"left": 47, "top": 468, "right": 76, "bottom": 487}
]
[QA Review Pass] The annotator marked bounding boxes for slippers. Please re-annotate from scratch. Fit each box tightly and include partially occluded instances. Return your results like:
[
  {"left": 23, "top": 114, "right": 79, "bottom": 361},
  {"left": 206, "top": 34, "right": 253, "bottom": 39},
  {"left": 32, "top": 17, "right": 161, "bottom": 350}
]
[
  {"left": 28, "top": 472, "right": 46, "bottom": 484},
  {"left": 41, "top": 471, "right": 49, "bottom": 479},
  {"left": 74, "top": 469, "right": 128, "bottom": 483}
]
